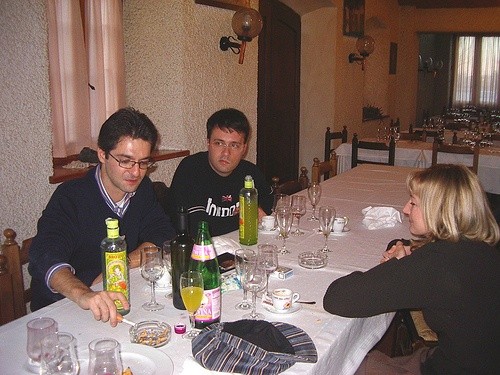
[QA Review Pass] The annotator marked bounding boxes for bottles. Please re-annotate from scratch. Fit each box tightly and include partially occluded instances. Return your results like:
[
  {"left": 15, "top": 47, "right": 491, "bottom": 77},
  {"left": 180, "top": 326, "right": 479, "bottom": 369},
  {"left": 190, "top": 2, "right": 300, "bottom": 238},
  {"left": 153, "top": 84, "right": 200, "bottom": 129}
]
[
  {"left": 100, "top": 218, "right": 131, "bottom": 317},
  {"left": 171, "top": 206, "right": 194, "bottom": 311},
  {"left": 188, "top": 219, "right": 222, "bottom": 328},
  {"left": 377, "top": 105, "right": 500, "bottom": 150},
  {"left": 239, "top": 175, "right": 259, "bottom": 246}
]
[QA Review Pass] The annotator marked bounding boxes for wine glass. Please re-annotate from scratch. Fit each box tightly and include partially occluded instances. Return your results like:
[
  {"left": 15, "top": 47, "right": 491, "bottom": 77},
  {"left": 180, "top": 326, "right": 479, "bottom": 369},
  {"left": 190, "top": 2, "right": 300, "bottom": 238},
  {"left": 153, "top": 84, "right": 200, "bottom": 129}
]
[
  {"left": 273, "top": 194, "right": 307, "bottom": 257},
  {"left": 139, "top": 245, "right": 166, "bottom": 312},
  {"left": 234, "top": 243, "right": 278, "bottom": 321},
  {"left": 318, "top": 206, "right": 337, "bottom": 254},
  {"left": 307, "top": 182, "right": 322, "bottom": 222},
  {"left": 162, "top": 239, "right": 173, "bottom": 301}
]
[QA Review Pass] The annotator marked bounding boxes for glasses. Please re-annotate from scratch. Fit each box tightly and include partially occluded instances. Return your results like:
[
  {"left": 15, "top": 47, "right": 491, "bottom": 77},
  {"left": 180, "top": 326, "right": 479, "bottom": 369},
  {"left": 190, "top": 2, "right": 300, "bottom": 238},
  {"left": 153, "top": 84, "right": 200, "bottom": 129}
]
[
  {"left": 209, "top": 141, "right": 246, "bottom": 153},
  {"left": 109, "top": 153, "right": 156, "bottom": 169}
]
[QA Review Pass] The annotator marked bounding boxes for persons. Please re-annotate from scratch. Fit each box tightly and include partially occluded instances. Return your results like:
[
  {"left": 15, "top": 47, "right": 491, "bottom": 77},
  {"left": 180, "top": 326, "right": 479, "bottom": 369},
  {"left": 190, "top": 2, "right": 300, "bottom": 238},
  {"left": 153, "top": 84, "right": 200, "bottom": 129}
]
[
  {"left": 26, "top": 109, "right": 176, "bottom": 326},
  {"left": 323, "top": 164, "right": 500, "bottom": 375},
  {"left": 171, "top": 109, "right": 277, "bottom": 238}
]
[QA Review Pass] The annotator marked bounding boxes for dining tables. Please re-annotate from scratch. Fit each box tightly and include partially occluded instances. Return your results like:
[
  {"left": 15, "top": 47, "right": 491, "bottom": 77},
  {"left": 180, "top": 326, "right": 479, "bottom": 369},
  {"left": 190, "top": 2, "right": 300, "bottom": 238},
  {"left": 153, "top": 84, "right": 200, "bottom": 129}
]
[
  {"left": 331, "top": 141, "right": 500, "bottom": 196},
  {"left": 0, "top": 163, "right": 431, "bottom": 375}
]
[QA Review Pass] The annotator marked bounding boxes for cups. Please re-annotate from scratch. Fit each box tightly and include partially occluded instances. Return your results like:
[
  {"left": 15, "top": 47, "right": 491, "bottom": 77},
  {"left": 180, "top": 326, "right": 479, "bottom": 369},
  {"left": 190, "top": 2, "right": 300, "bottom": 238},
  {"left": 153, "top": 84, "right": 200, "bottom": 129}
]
[
  {"left": 25, "top": 317, "right": 58, "bottom": 374},
  {"left": 39, "top": 332, "right": 80, "bottom": 375},
  {"left": 332, "top": 216, "right": 350, "bottom": 232},
  {"left": 262, "top": 215, "right": 276, "bottom": 231},
  {"left": 88, "top": 337, "right": 123, "bottom": 375},
  {"left": 273, "top": 289, "right": 300, "bottom": 311}
]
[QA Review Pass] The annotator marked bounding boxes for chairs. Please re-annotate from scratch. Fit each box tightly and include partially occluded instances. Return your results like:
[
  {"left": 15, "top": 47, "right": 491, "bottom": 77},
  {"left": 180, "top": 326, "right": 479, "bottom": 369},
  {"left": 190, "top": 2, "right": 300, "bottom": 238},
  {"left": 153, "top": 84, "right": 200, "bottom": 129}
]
[
  {"left": 351, "top": 131, "right": 396, "bottom": 167},
  {"left": 0, "top": 225, "right": 33, "bottom": 326},
  {"left": 312, "top": 150, "right": 340, "bottom": 184},
  {"left": 432, "top": 137, "right": 479, "bottom": 173},
  {"left": 272, "top": 166, "right": 309, "bottom": 200},
  {"left": 324, "top": 123, "right": 349, "bottom": 180},
  {"left": 396, "top": 117, "right": 427, "bottom": 143},
  {"left": 452, "top": 132, "right": 481, "bottom": 145}
]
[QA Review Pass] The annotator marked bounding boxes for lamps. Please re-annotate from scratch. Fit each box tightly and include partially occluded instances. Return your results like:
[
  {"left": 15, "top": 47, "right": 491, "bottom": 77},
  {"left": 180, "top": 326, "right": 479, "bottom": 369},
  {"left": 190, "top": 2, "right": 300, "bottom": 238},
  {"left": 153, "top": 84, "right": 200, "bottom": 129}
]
[
  {"left": 419, "top": 49, "right": 435, "bottom": 71},
  {"left": 219, "top": 6, "right": 263, "bottom": 64},
  {"left": 349, "top": 35, "right": 376, "bottom": 71}
]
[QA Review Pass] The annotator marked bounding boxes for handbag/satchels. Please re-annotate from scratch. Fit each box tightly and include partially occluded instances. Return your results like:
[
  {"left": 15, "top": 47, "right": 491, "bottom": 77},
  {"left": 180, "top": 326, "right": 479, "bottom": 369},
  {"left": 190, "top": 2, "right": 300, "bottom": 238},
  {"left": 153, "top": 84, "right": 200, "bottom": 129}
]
[{"left": 374, "top": 310, "right": 415, "bottom": 357}]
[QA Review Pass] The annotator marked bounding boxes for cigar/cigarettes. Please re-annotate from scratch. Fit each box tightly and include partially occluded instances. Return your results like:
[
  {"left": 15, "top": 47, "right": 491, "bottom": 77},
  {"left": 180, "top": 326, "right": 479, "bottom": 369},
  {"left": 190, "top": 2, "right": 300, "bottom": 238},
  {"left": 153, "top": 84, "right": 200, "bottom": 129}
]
[{"left": 116, "top": 317, "right": 136, "bottom": 326}]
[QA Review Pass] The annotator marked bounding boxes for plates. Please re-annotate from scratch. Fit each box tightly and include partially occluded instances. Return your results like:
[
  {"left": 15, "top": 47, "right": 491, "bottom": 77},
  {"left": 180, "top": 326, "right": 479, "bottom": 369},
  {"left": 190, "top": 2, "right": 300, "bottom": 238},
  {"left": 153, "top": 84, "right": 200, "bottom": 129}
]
[
  {"left": 330, "top": 227, "right": 351, "bottom": 235},
  {"left": 78, "top": 343, "right": 175, "bottom": 375},
  {"left": 263, "top": 298, "right": 303, "bottom": 315},
  {"left": 258, "top": 224, "right": 279, "bottom": 234}
]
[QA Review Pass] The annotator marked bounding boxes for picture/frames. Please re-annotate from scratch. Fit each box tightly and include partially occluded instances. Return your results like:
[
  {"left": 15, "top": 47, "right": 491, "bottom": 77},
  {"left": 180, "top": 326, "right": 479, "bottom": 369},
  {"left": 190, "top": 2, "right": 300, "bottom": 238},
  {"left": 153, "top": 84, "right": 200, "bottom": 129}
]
[{"left": 342, "top": 0, "right": 398, "bottom": 75}]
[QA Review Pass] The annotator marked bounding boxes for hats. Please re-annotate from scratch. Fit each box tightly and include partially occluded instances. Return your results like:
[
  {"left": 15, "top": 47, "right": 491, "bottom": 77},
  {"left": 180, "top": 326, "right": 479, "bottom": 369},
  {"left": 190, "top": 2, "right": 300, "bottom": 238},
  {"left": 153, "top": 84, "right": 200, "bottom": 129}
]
[{"left": 191, "top": 319, "right": 318, "bottom": 375}]
